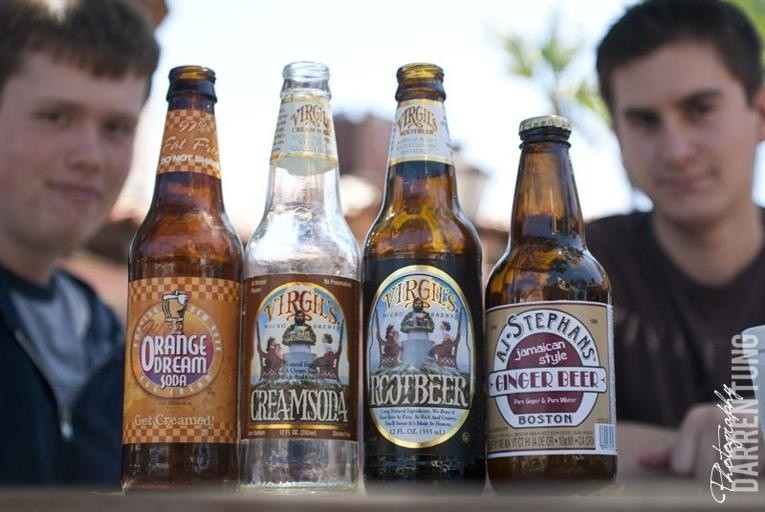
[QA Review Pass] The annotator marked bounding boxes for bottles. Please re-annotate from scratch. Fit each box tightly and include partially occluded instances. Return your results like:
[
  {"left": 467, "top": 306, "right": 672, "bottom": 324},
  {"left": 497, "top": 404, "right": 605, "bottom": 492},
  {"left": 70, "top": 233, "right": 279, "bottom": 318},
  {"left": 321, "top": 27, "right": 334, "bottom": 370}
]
[
  {"left": 239, "top": 61, "right": 364, "bottom": 493},
  {"left": 362, "top": 62, "right": 484, "bottom": 494},
  {"left": 485, "top": 116, "right": 618, "bottom": 493},
  {"left": 122, "top": 65, "right": 243, "bottom": 493}
]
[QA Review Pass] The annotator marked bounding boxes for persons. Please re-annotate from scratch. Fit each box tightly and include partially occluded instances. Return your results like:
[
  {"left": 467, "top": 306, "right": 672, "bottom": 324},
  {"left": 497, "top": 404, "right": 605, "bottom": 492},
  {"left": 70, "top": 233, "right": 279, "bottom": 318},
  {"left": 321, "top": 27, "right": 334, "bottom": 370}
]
[
  {"left": 585, "top": 1, "right": 765, "bottom": 481},
  {"left": 1, "top": 1, "right": 161, "bottom": 482}
]
